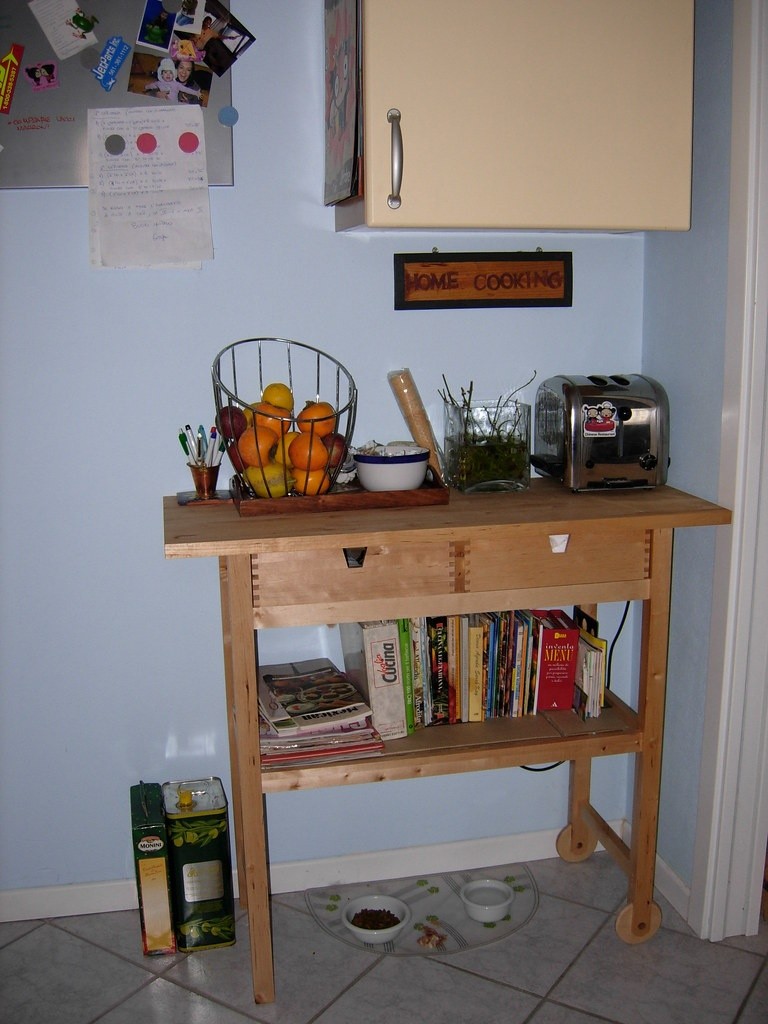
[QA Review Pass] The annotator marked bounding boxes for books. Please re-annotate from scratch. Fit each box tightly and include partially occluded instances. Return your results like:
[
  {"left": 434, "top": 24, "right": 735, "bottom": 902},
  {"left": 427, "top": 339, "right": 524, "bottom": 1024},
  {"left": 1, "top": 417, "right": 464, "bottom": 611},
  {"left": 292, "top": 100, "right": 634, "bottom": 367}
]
[{"left": 258, "top": 609, "right": 609, "bottom": 771}]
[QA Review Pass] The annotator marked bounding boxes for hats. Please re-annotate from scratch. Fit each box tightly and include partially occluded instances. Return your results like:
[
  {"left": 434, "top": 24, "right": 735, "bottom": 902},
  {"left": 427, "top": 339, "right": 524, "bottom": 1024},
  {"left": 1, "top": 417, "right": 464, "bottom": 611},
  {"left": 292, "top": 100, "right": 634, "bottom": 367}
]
[{"left": 158, "top": 58, "right": 176, "bottom": 82}]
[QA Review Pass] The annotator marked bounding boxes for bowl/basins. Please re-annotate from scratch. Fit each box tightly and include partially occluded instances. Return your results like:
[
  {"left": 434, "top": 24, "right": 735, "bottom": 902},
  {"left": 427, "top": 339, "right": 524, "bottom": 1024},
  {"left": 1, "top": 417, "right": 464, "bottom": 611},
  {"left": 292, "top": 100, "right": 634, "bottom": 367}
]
[
  {"left": 460, "top": 879, "right": 514, "bottom": 923},
  {"left": 353, "top": 446, "right": 430, "bottom": 492},
  {"left": 341, "top": 895, "right": 411, "bottom": 944}
]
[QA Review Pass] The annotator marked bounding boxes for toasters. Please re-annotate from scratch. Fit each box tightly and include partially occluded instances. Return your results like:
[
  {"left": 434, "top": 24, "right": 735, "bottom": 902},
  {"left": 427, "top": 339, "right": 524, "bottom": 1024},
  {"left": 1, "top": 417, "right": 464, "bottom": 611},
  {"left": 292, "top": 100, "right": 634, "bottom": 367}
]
[{"left": 530, "top": 374, "right": 671, "bottom": 495}]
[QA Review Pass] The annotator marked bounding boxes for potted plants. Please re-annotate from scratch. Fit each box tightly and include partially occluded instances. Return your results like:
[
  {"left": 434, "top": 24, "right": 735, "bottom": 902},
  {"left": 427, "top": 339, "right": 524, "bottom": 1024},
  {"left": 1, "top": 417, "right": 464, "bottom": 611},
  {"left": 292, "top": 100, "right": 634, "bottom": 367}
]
[{"left": 442, "top": 400, "right": 535, "bottom": 495}]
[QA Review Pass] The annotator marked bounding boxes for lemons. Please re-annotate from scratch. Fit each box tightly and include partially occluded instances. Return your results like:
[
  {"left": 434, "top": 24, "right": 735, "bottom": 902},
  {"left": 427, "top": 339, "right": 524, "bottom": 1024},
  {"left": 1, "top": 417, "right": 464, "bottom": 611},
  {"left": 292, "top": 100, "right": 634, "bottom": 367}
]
[{"left": 243, "top": 382, "right": 304, "bottom": 498}]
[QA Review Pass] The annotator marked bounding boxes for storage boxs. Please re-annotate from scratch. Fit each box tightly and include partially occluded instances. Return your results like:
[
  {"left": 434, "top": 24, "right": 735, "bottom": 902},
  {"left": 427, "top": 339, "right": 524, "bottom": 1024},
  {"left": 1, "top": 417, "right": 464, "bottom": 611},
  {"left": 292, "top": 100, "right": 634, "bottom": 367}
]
[{"left": 130, "top": 782, "right": 175, "bottom": 959}]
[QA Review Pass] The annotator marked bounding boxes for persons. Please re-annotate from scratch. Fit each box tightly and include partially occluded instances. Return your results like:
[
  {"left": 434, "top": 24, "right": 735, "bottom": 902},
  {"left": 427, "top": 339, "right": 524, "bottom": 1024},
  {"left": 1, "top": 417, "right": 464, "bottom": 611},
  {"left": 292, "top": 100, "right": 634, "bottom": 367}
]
[{"left": 139, "top": 1, "right": 240, "bottom": 106}]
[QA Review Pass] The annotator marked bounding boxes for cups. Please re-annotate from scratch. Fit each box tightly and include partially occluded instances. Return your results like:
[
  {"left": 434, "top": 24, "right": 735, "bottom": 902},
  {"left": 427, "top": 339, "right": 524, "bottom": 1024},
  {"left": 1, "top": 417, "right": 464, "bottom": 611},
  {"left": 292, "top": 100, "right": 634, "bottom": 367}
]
[{"left": 186, "top": 462, "right": 221, "bottom": 497}]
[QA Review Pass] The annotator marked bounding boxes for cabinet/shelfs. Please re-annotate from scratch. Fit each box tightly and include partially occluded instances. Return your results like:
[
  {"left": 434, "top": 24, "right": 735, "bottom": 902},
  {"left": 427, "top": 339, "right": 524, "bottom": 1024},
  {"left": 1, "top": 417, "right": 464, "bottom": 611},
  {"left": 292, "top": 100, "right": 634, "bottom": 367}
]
[{"left": 332, "top": 0, "right": 695, "bottom": 234}]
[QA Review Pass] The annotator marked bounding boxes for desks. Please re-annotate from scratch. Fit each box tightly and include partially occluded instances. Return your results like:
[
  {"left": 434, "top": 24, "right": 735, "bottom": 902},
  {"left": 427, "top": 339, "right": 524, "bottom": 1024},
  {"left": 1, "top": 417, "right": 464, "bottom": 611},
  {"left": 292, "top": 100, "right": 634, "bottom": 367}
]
[{"left": 160, "top": 490, "right": 732, "bottom": 1006}]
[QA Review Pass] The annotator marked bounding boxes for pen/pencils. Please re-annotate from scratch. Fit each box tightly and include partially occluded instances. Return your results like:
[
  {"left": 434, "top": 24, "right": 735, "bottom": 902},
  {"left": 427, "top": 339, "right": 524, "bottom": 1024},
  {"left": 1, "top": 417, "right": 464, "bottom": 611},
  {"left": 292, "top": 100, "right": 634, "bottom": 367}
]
[{"left": 179, "top": 424, "right": 227, "bottom": 466}]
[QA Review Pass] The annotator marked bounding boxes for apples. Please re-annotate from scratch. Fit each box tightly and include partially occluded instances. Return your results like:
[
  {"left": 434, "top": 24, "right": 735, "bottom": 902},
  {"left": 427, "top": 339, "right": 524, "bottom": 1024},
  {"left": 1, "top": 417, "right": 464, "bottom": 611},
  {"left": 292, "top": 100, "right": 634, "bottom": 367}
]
[
  {"left": 214, "top": 405, "right": 250, "bottom": 474},
  {"left": 321, "top": 432, "right": 348, "bottom": 467}
]
